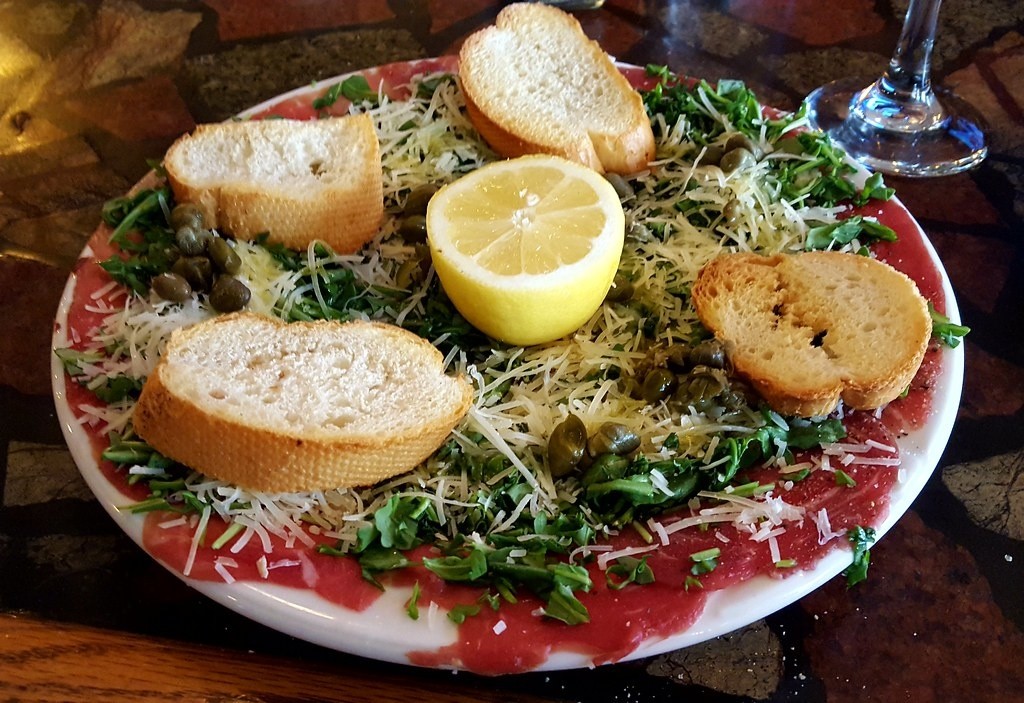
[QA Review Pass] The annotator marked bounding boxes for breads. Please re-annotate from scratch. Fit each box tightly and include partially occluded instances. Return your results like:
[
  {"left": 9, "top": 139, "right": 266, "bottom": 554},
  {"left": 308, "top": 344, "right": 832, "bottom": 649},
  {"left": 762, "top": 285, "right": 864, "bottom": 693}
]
[
  {"left": 131, "top": 311, "right": 471, "bottom": 493},
  {"left": 161, "top": 111, "right": 384, "bottom": 258},
  {"left": 454, "top": 1, "right": 656, "bottom": 177},
  {"left": 690, "top": 248, "right": 934, "bottom": 419}
]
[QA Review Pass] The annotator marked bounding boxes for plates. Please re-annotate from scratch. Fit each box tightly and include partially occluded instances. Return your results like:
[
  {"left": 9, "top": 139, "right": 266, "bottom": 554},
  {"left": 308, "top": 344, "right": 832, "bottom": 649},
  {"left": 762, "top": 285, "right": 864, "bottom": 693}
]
[{"left": 47, "top": 54, "right": 964, "bottom": 678}]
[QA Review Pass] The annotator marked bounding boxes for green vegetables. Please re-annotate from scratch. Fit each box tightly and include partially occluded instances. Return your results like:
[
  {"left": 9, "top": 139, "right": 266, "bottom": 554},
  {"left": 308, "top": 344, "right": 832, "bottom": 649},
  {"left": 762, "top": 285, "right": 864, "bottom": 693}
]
[{"left": 60, "top": 65, "right": 970, "bottom": 623}]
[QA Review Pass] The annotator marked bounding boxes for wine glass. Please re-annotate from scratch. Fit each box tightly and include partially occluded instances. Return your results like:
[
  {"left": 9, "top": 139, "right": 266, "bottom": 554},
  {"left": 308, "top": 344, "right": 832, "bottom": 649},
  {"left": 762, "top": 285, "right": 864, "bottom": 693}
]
[{"left": 800, "top": 0, "right": 993, "bottom": 178}]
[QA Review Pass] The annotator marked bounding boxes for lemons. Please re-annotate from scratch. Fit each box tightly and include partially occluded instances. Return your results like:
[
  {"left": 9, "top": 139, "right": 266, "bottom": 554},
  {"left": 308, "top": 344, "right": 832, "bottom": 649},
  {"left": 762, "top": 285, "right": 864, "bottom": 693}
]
[{"left": 426, "top": 152, "right": 625, "bottom": 345}]
